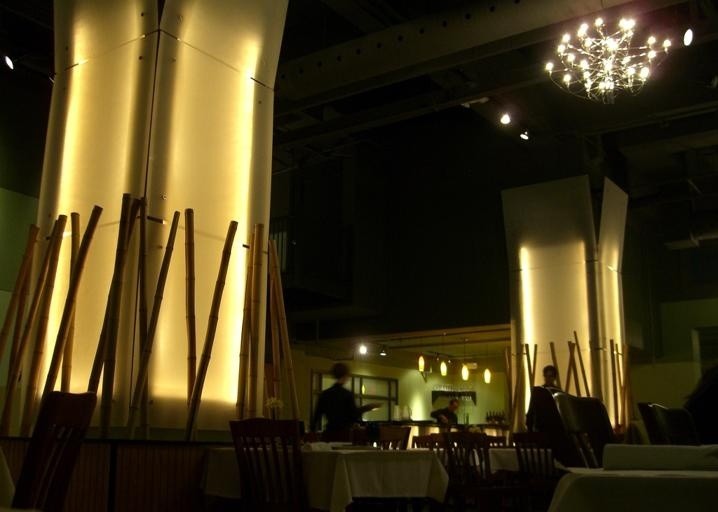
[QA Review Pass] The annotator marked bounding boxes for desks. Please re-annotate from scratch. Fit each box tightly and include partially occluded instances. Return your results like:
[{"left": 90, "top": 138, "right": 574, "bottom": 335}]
[{"left": 549, "top": 469, "right": 718, "bottom": 512}]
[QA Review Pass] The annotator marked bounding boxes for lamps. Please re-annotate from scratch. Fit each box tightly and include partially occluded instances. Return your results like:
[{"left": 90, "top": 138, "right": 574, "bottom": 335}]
[
  {"left": 544, "top": 1, "right": 672, "bottom": 107},
  {"left": 415, "top": 331, "right": 491, "bottom": 388}
]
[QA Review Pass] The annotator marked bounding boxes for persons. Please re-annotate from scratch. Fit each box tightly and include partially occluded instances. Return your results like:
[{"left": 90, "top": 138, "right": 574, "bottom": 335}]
[
  {"left": 526, "top": 366, "right": 562, "bottom": 433},
  {"left": 309, "top": 363, "right": 381, "bottom": 442},
  {"left": 431, "top": 399, "right": 459, "bottom": 425}
]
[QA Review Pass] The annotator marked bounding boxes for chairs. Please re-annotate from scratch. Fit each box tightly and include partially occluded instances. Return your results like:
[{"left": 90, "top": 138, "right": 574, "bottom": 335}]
[
  {"left": 371, "top": 427, "right": 411, "bottom": 449},
  {"left": 228, "top": 418, "right": 308, "bottom": 512},
  {"left": 533, "top": 386, "right": 622, "bottom": 467},
  {"left": 13, "top": 391, "right": 96, "bottom": 512},
  {"left": 412, "top": 432, "right": 558, "bottom": 512},
  {"left": 637, "top": 402, "right": 703, "bottom": 446}
]
